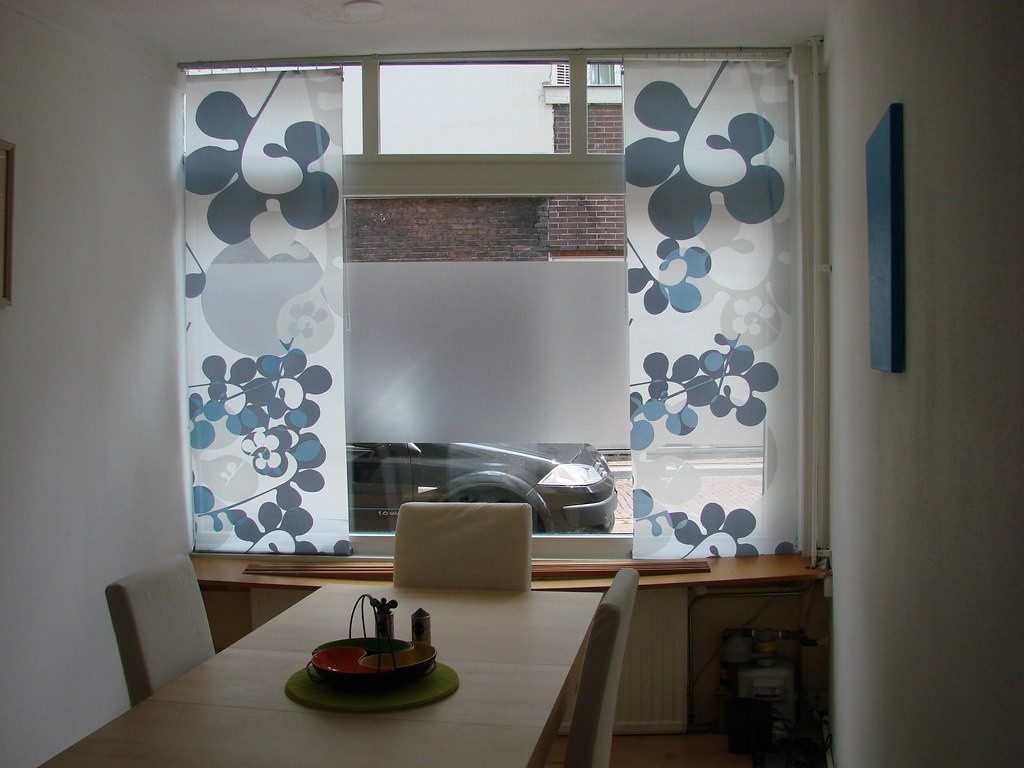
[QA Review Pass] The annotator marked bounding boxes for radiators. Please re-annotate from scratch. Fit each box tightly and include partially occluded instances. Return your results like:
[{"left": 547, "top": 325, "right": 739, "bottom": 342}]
[{"left": 250, "top": 588, "right": 688, "bottom": 735}]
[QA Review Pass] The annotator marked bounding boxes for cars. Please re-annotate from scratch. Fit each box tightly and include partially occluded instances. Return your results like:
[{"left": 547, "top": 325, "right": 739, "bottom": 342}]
[{"left": 347, "top": 441, "right": 621, "bottom": 534}]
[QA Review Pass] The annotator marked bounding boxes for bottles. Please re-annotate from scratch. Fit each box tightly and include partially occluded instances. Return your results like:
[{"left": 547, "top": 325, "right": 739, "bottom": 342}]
[
  {"left": 411, "top": 607, "right": 431, "bottom": 645},
  {"left": 374, "top": 609, "right": 394, "bottom": 638}
]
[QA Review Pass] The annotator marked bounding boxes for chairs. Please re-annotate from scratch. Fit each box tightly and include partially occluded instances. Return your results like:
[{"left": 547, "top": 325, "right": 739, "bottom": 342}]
[
  {"left": 105, "top": 552, "right": 215, "bottom": 709},
  {"left": 564, "top": 568, "right": 639, "bottom": 768},
  {"left": 393, "top": 502, "right": 533, "bottom": 591}
]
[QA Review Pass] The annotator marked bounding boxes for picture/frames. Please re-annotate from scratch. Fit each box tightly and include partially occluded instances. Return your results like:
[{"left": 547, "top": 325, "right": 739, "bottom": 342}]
[{"left": 0, "top": 139, "right": 16, "bottom": 306}]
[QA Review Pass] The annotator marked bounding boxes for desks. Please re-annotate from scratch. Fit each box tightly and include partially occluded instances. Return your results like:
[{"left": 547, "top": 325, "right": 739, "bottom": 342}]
[{"left": 39, "top": 584, "right": 605, "bottom": 768}]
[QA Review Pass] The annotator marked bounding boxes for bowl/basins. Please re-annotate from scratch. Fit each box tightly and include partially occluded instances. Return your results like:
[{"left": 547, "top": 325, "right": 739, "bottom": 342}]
[{"left": 311, "top": 638, "right": 437, "bottom": 692}]
